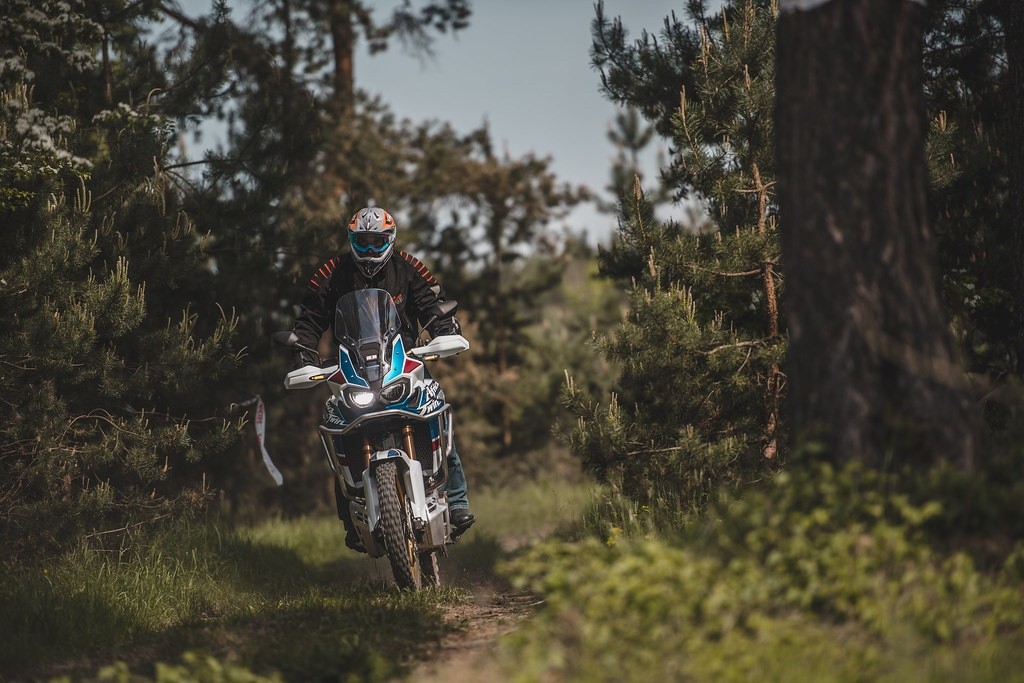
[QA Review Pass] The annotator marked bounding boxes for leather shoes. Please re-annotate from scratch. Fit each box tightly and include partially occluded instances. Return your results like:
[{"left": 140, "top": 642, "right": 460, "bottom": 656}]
[
  {"left": 344, "top": 530, "right": 361, "bottom": 547},
  {"left": 450, "top": 508, "right": 476, "bottom": 529}
]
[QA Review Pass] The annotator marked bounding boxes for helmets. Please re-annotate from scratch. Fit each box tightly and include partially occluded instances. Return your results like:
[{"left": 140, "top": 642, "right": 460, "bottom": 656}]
[{"left": 346, "top": 206, "right": 396, "bottom": 279}]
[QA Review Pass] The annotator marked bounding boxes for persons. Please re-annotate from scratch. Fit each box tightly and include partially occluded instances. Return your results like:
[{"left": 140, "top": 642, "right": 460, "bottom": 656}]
[{"left": 292, "top": 208, "right": 476, "bottom": 549}]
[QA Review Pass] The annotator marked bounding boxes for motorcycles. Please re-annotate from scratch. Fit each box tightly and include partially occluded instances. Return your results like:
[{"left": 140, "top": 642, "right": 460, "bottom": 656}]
[{"left": 271, "top": 288, "right": 477, "bottom": 597}]
